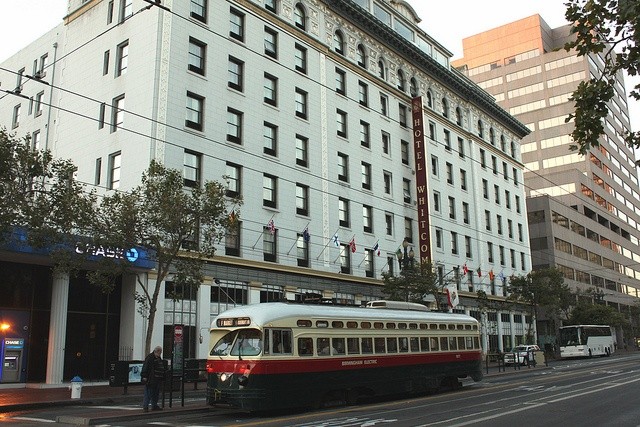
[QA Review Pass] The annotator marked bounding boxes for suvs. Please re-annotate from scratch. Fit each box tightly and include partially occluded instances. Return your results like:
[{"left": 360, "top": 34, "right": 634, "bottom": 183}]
[{"left": 504, "top": 345, "right": 540, "bottom": 366}]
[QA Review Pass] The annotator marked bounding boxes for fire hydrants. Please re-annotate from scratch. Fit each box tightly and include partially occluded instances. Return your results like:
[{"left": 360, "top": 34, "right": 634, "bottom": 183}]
[{"left": 69, "top": 376, "right": 83, "bottom": 398}]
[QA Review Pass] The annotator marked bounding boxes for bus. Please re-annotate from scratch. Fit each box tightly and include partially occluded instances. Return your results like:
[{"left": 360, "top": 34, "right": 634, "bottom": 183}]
[
  {"left": 206, "top": 300, "right": 484, "bottom": 411},
  {"left": 559, "top": 325, "right": 615, "bottom": 359}
]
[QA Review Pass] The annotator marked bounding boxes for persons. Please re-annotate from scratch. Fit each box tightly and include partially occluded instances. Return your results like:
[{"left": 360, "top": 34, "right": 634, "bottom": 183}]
[{"left": 141, "top": 346, "right": 169, "bottom": 411}]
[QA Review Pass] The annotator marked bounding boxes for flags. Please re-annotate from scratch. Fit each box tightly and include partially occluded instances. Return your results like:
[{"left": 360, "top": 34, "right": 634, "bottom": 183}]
[
  {"left": 396, "top": 249, "right": 403, "bottom": 261},
  {"left": 332, "top": 233, "right": 340, "bottom": 248},
  {"left": 373, "top": 243, "right": 380, "bottom": 256},
  {"left": 445, "top": 281, "right": 460, "bottom": 309},
  {"left": 462, "top": 264, "right": 468, "bottom": 275},
  {"left": 303, "top": 227, "right": 310, "bottom": 241},
  {"left": 476, "top": 267, "right": 482, "bottom": 277},
  {"left": 349, "top": 239, "right": 356, "bottom": 253},
  {"left": 489, "top": 271, "right": 494, "bottom": 280},
  {"left": 229, "top": 211, "right": 235, "bottom": 225},
  {"left": 498, "top": 271, "right": 504, "bottom": 282},
  {"left": 267, "top": 220, "right": 276, "bottom": 235}
]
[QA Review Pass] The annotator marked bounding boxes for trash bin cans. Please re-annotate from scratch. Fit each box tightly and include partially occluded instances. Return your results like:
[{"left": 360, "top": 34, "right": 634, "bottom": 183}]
[{"left": 545, "top": 343, "right": 553, "bottom": 352}]
